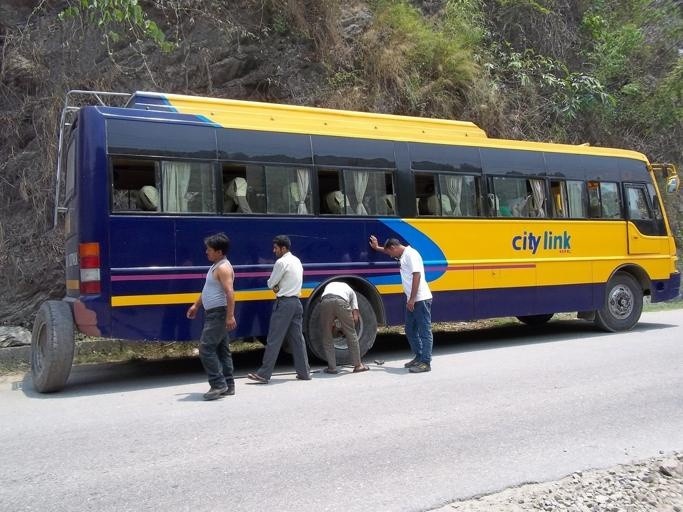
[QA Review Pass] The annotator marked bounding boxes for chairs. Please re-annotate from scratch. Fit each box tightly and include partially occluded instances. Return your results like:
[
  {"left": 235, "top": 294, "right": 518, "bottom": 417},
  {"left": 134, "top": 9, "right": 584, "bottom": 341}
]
[
  {"left": 282, "top": 181, "right": 300, "bottom": 213},
  {"left": 488, "top": 193, "right": 500, "bottom": 216},
  {"left": 377, "top": 195, "right": 396, "bottom": 215},
  {"left": 364, "top": 195, "right": 375, "bottom": 214},
  {"left": 428, "top": 194, "right": 462, "bottom": 216},
  {"left": 226, "top": 177, "right": 253, "bottom": 214},
  {"left": 143, "top": 185, "right": 158, "bottom": 212},
  {"left": 327, "top": 190, "right": 366, "bottom": 213}
]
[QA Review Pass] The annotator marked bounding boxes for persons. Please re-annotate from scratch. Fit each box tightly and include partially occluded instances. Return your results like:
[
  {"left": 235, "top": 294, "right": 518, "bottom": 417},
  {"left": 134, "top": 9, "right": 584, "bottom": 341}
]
[
  {"left": 186, "top": 232, "right": 238, "bottom": 400},
  {"left": 369, "top": 235, "right": 434, "bottom": 373},
  {"left": 317, "top": 281, "right": 370, "bottom": 373},
  {"left": 247, "top": 234, "right": 312, "bottom": 383}
]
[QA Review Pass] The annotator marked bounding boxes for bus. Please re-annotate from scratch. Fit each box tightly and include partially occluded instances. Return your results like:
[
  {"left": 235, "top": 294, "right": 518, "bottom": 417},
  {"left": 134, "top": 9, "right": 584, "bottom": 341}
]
[{"left": 52, "top": 89, "right": 682, "bottom": 364}]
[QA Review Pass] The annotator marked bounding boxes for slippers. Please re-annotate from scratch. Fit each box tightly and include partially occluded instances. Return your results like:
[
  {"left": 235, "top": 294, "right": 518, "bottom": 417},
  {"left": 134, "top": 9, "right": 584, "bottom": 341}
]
[
  {"left": 354, "top": 366, "right": 369, "bottom": 372},
  {"left": 248, "top": 373, "right": 270, "bottom": 383},
  {"left": 324, "top": 368, "right": 337, "bottom": 374}
]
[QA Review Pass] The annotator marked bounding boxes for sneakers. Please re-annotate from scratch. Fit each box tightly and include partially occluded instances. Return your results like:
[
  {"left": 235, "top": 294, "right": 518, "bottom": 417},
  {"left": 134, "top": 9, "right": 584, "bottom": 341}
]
[
  {"left": 405, "top": 360, "right": 420, "bottom": 367},
  {"left": 410, "top": 362, "right": 431, "bottom": 373},
  {"left": 204, "top": 385, "right": 235, "bottom": 400}
]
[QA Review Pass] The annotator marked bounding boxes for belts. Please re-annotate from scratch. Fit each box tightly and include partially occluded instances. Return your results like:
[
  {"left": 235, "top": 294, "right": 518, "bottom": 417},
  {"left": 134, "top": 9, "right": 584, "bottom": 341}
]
[{"left": 204, "top": 306, "right": 227, "bottom": 314}]
